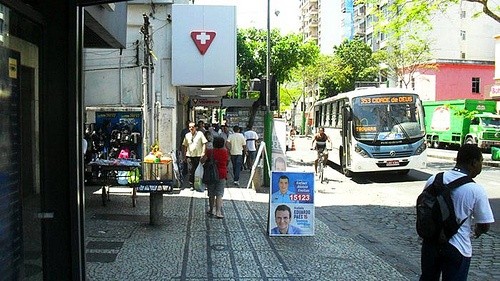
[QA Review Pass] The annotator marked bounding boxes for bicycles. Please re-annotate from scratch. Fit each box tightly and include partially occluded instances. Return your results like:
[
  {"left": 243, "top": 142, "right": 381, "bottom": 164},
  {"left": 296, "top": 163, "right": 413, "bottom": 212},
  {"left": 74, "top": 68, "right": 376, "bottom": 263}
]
[{"left": 310, "top": 147, "right": 332, "bottom": 184}]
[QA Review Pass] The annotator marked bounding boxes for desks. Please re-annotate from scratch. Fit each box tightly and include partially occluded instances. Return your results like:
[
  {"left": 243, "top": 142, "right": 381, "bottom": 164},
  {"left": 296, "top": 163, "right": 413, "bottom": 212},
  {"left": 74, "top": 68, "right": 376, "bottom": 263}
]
[{"left": 142, "top": 161, "right": 175, "bottom": 181}]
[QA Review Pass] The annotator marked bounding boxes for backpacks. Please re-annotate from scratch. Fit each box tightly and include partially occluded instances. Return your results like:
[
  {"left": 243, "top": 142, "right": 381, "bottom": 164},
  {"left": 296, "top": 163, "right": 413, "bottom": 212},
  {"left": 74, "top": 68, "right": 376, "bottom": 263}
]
[{"left": 415, "top": 172, "right": 476, "bottom": 244}]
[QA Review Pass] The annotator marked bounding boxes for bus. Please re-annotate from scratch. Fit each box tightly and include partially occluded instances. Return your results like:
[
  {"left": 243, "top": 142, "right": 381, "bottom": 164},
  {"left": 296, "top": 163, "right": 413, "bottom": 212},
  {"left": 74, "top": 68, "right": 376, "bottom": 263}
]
[{"left": 310, "top": 86, "right": 428, "bottom": 178}]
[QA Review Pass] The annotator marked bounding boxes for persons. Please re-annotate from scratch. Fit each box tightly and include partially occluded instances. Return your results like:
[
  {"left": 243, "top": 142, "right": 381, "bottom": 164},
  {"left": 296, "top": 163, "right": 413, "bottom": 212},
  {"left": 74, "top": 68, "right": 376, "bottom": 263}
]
[
  {"left": 416, "top": 144, "right": 495, "bottom": 281},
  {"left": 272, "top": 205, "right": 303, "bottom": 234},
  {"left": 185, "top": 120, "right": 262, "bottom": 185},
  {"left": 272, "top": 175, "right": 299, "bottom": 203},
  {"left": 311, "top": 127, "right": 329, "bottom": 175},
  {"left": 182, "top": 122, "right": 208, "bottom": 191},
  {"left": 391, "top": 109, "right": 410, "bottom": 126},
  {"left": 201, "top": 137, "right": 229, "bottom": 218}
]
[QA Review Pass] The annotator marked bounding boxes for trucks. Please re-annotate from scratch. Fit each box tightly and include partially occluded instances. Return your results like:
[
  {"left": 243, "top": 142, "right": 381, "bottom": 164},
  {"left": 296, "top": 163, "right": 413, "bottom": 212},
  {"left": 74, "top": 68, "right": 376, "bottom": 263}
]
[{"left": 418, "top": 98, "right": 500, "bottom": 153}]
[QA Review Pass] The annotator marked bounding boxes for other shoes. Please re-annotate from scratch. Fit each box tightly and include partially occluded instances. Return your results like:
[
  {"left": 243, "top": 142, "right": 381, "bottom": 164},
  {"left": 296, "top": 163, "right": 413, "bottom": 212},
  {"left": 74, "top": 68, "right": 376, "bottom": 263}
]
[{"left": 234, "top": 180, "right": 240, "bottom": 185}]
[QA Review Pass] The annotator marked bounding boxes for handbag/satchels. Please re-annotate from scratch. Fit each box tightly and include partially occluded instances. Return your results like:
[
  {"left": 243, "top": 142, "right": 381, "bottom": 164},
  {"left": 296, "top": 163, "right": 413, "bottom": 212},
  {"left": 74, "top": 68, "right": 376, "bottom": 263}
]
[
  {"left": 193, "top": 160, "right": 206, "bottom": 192},
  {"left": 201, "top": 149, "right": 219, "bottom": 184}
]
